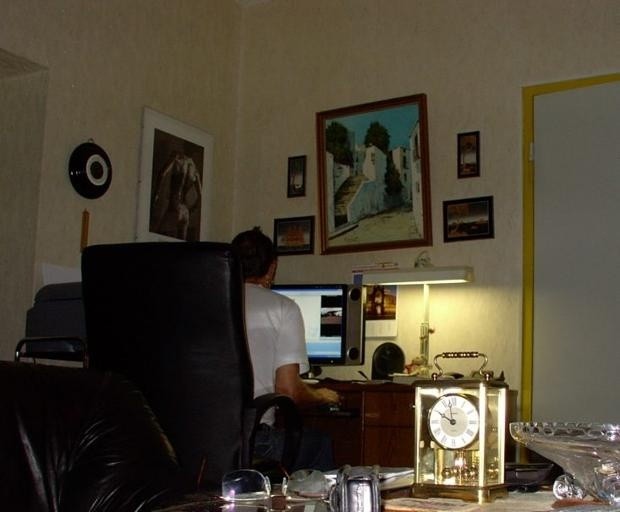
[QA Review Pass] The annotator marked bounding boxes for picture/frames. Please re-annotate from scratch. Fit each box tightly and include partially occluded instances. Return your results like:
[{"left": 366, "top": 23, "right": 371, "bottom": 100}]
[
  {"left": 274, "top": 215, "right": 313, "bottom": 254},
  {"left": 457, "top": 130, "right": 481, "bottom": 177},
  {"left": 288, "top": 155, "right": 307, "bottom": 197},
  {"left": 137, "top": 106, "right": 214, "bottom": 243},
  {"left": 443, "top": 196, "right": 494, "bottom": 241},
  {"left": 315, "top": 92, "right": 432, "bottom": 253}
]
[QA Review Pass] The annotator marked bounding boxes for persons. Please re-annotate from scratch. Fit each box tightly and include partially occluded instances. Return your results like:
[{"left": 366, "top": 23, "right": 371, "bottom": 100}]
[{"left": 230, "top": 226, "right": 345, "bottom": 475}]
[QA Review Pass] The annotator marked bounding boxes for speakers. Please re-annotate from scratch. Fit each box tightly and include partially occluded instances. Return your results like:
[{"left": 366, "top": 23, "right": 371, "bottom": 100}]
[{"left": 346, "top": 285, "right": 367, "bottom": 366}]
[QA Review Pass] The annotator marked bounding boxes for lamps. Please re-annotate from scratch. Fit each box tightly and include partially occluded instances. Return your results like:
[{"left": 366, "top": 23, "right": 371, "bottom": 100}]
[{"left": 361, "top": 260, "right": 475, "bottom": 375}]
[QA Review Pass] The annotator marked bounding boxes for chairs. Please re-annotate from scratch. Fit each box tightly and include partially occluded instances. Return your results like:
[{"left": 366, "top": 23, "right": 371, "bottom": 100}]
[
  {"left": 0, "top": 363, "right": 212, "bottom": 511},
  {"left": 82, "top": 239, "right": 303, "bottom": 487}
]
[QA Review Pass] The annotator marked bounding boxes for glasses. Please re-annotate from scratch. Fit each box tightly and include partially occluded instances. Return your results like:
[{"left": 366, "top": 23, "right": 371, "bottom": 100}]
[{"left": 197, "top": 458, "right": 330, "bottom": 502}]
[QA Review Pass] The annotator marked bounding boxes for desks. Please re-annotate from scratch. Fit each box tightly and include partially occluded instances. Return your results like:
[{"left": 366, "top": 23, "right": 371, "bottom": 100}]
[{"left": 165, "top": 467, "right": 595, "bottom": 512}]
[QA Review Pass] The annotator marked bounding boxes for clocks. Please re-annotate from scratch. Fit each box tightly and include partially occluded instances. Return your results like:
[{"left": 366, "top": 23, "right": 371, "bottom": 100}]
[{"left": 410, "top": 351, "right": 510, "bottom": 504}]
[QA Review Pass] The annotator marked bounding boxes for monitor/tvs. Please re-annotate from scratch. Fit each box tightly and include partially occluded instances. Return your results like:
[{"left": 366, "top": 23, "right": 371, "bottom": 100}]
[{"left": 271, "top": 284, "right": 347, "bottom": 378}]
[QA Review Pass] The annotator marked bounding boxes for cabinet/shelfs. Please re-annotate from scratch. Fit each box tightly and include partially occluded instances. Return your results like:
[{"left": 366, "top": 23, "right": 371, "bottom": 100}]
[{"left": 306, "top": 376, "right": 415, "bottom": 462}]
